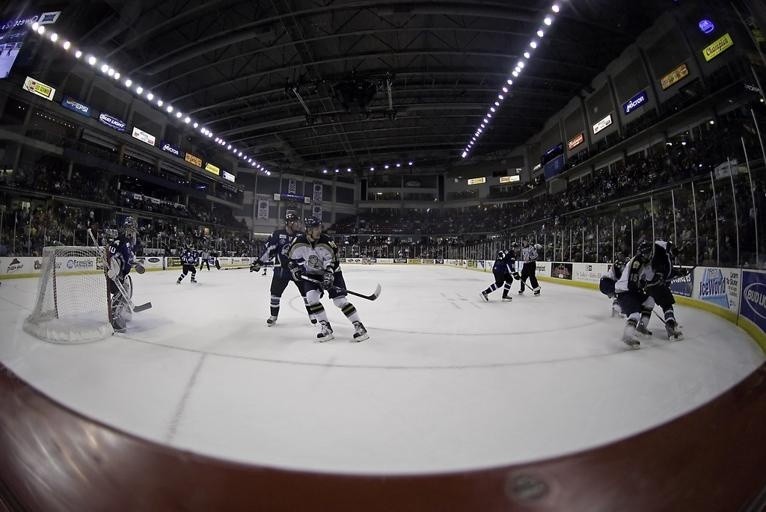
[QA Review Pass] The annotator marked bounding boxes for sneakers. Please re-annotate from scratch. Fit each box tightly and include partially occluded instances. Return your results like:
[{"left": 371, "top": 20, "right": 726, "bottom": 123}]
[
  {"left": 501, "top": 295, "right": 513, "bottom": 300},
  {"left": 309, "top": 313, "right": 318, "bottom": 324},
  {"left": 518, "top": 290, "right": 523, "bottom": 294},
  {"left": 481, "top": 290, "right": 489, "bottom": 303},
  {"left": 612, "top": 301, "right": 627, "bottom": 318},
  {"left": 622, "top": 320, "right": 685, "bottom": 347},
  {"left": 316, "top": 319, "right": 334, "bottom": 339},
  {"left": 176, "top": 279, "right": 197, "bottom": 284},
  {"left": 351, "top": 320, "right": 367, "bottom": 339},
  {"left": 533, "top": 288, "right": 541, "bottom": 295},
  {"left": 266, "top": 315, "right": 278, "bottom": 324}
]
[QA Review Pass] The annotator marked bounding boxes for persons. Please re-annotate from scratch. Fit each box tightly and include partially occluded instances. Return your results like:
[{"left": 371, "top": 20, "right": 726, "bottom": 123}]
[
  {"left": 518, "top": 237, "right": 542, "bottom": 297},
  {"left": 106, "top": 215, "right": 148, "bottom": 330},
  {"left": 200, "top": 248, "right": 211, "bottom": 270},
  {"left": 176, "top": 245, "right": 200, "bottom": 284},
  {"left": 520, "top": 129, "right": 765, "bottom": 272},
  {"left": 250, "top": 211, "right": 319, "bottom": 324},
  {"left": 600, "top": 238, "right": 684, "bottom": 350},
  {"left": 287, "top": 216, "right": 366, "bottom": 338},
  {"left": 0, "top": 46, "right": 13, "bottom": 78},
  {"left": 0, "top": 169, "right": 272, "bottom": 257},
  {"left": 482, "top": 241, "right": 521, "bottom": 301},
  {"left": 325, "top": 202, "right": 520, "bottom": 260}
]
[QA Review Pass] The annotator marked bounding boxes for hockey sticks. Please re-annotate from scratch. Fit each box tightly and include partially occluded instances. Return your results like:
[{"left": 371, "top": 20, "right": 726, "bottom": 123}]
[
  {"left": 518, "top": 277, "right": 541, "bottom": 292},
  {"left": 87, "top": 228, "right": 152, "bottom": 312},
  {"left": 299, "top": 275, "right": 381, "bottom": 300}
]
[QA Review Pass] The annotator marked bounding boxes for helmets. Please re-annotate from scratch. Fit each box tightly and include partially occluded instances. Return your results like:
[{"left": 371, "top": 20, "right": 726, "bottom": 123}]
[
  {"left": 636, "top": 240, "right": 653, "bottom": 256},
  {"left": 303, "top": 215, "right": 323, "bottom": 228},
  {"left": 284, "top": 213, "right": 298, "bottom": 223},
  {"left": 522, "top": 236, "right": 530, "bottom": 242},
  {"left": 123, "top": 215, "right": 139, "bottom": 233},
  {"left": 510, "top": 241, "right": 521, "bottom": 248}
]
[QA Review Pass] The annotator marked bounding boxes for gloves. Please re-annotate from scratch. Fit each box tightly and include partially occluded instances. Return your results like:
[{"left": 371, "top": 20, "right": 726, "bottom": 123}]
[
  {"left": 286, "top": 260, "right": 307, "bottom": 283},
  {"left": 319, "top": 269, "right": 335, "bottom": 291},
  {"left": 249, "top": 259, "right": 264, "bottom": 273}
]
